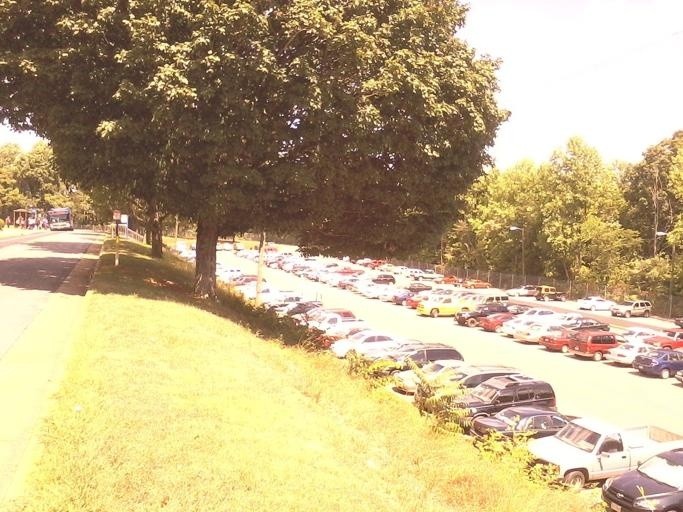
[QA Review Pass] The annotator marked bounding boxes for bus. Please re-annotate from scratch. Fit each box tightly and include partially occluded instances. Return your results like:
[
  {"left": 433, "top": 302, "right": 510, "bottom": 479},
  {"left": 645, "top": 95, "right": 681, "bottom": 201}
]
[{"left": 46, "top": 207, "right": 73, "bottom": 231}]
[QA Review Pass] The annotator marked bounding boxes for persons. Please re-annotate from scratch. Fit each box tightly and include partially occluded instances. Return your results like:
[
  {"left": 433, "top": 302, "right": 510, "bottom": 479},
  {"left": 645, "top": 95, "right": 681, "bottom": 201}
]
[{"left": 5, "top": 214, "right": 48, "bottom": 229}]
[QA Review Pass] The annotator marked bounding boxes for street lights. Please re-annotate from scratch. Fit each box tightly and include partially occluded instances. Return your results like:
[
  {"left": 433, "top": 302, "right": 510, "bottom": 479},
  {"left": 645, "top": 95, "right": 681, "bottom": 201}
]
[
  {"left": 656, "top": 230, "right": 676, "bottom": 318},
  {"left": 509, "top": 226, "right": 525, "bottom": 276}
]
